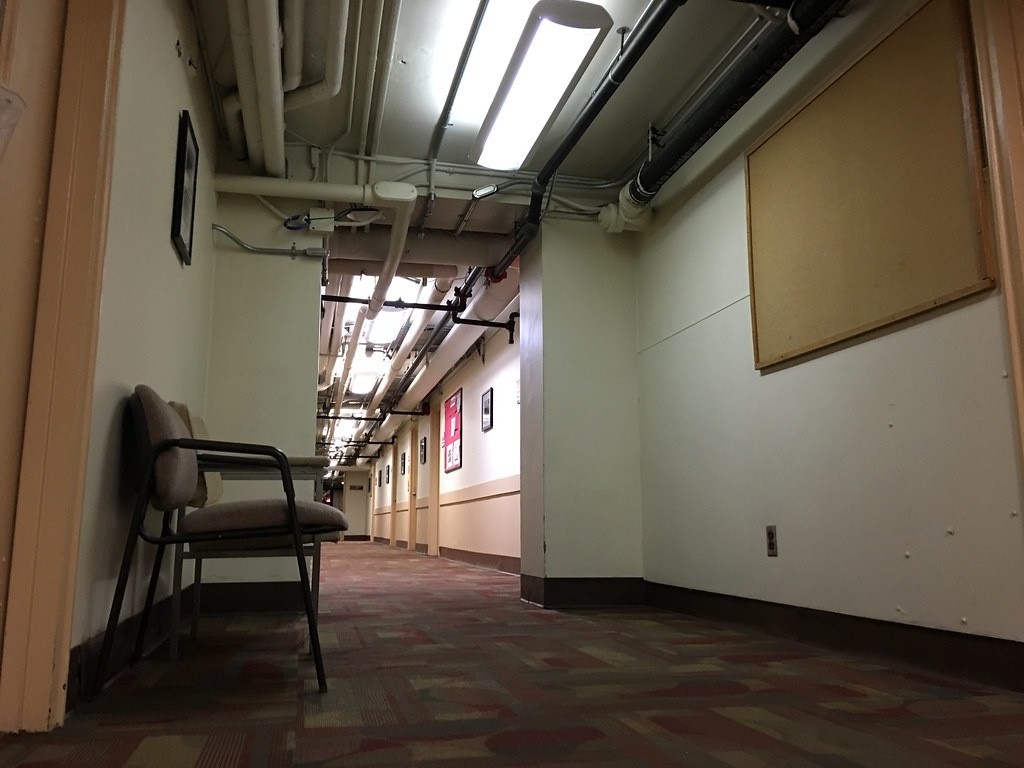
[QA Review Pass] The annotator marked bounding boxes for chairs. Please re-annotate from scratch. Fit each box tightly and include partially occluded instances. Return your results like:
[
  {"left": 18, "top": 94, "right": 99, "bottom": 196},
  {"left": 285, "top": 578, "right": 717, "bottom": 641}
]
[{"left": 85, "top": 382, "right": 347, "bottom": 694}]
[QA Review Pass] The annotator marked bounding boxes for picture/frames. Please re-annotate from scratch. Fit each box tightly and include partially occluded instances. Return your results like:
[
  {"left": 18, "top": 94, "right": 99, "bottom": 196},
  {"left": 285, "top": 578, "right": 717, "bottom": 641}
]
[
  {"left": 480, "top": 388, "right": 494, "bottom": 433},
  {"left": 171, "top": 110, "right": 200, "bottom": 267}
]
